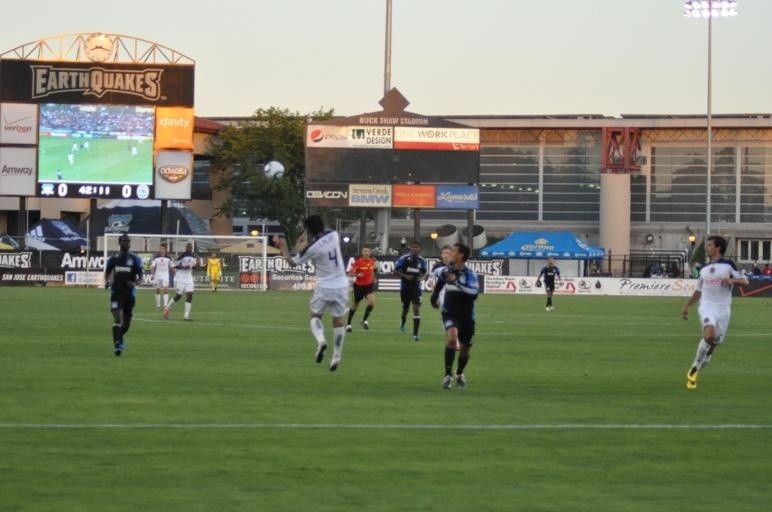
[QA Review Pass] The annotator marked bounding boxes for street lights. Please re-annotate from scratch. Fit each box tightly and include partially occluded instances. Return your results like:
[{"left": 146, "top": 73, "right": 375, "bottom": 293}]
[
  {"left": 681, "top": 0, "right": 741, "bottom": 267},
  {"left": 688, "top": 234, "right": 696, "bottom": 250},
  {"left": 431, "top": 230, "right": 438, "bottom": 257}
]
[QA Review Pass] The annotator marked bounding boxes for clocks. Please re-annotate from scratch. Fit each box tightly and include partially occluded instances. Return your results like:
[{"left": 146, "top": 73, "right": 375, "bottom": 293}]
[{"left": 83, "top": 33, "right": 114, "bottom": 63}]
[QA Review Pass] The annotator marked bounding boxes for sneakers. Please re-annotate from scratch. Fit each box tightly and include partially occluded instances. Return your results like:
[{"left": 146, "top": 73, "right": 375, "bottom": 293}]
[
  {"left": 442, "top": 371, "right": 466, "bottom": 389},
  {"left": 686, "top": 367, "right": 698, "bottom": 388},
  {"left": 346, "top": 320, "right": 369, "bottom": 332},
  {"left": 315, "top": 342, "right": 339, "bottom": 371}
]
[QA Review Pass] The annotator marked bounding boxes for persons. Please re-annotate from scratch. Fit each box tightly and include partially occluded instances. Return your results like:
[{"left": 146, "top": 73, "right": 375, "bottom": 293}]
[
  {"left": 270, "top": 214, "right": 352, "bottom": 372},
  {"left": 150, "top": 241, "right": 170, "bottom": 311},
  {"left": 39, "top": 102, "right": 156, "bottom": 185},
  {"left": 393, "top": 241, "right": 430, "bottom": 342},
  {"left": 206, "top": 251, "right": 224, "bottom": 291},
  {"left": 678, "top": 232, "right": 749, "bottom": 390},
  {"left": 536, "top": 256, "right": 566, "bottom": 312},
  {"left": 163, "top": 241, "right": 202, "bottom": 320},
  {"left": 425, "top": 244, "right": 460, "bottom": 351},
  {"left": 344, "top": 246, "right": 379, "bottom": 332},
  {"left": 584, "top": 252, "right": 771, "bottom": 286},
  {"left": 105, "top": 234, "right": 142, "bottom": 357},
  {"left": 425, "top": 242, "right": 480, "bottom": 390}
]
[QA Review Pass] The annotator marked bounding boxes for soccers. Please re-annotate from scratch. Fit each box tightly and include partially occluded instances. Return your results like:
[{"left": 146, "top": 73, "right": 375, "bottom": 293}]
[{"left": 265, "top": 162, "right": 283, "bottom": 180}]
